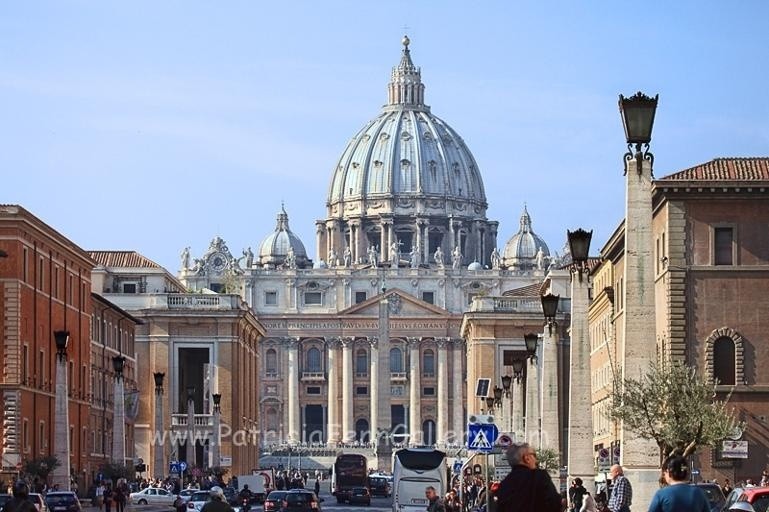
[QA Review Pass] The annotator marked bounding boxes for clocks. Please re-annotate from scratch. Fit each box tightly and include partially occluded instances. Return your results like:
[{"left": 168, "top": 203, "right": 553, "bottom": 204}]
[{"left": 207, "top": 251, "right": 229, "bottom": 273}]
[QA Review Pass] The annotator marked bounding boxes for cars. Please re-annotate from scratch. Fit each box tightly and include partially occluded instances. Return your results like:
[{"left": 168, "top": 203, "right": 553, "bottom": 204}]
[
  {"left": 687, "top": 482, "right": 769, "bottom": 512},
  {"left": 263, "top": 488, "right": 324, "bottom": 512},
  {"left": 349, "top": 472, "right": 393, "bottom": 506},
  {"left": 128, "top": 484, "right": 236, "bottom": 512},
  {"left": 0, "top": 490, "right": 81, "bottom": 512}
]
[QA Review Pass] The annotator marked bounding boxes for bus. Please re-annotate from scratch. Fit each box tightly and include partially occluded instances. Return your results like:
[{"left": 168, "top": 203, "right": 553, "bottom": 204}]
[
  {"left": 388, "top": 446, "right": 451, "bottom": 511},
  {"left": 328, "top": 454, "right": 366, "bottom": 502}
]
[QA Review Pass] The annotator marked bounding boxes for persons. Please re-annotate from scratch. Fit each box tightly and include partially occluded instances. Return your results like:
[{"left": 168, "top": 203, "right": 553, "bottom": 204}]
[
  {"left": 424, "top": 462, "right": 768, "bottom": 512},
  {"left": 494, "top": 440, "right": 565, "bottom": 512},
  {"left": 178, "top": 242, "right": 549, "bottom": 277},
  {"left": 1, "top": 456, "right": 328, "bottom": 512},
  {"left": 648, "top": 454, "right": 712, "bottom": 512}
]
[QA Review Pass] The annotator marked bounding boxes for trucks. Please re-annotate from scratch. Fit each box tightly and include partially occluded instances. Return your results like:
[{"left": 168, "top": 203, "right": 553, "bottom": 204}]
[{"left": 237, "top": 474, "right": 267, "bottom": 505}]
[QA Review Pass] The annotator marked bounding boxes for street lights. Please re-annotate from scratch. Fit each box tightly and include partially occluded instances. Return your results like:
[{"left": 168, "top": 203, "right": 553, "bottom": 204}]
[
  {"left": 483, "top": 356, "right": 524, "bottom": 440},
  {"left": 524, "top": 333, "right": 539, "bottom": 452},
  {"left": 212, "top": 393, "right": 222, "bottom": 468},
  {"left": 187, "top": 383, "right": 196, "bottom": 467},
  {"left": 567, "top": 228, "right": 591, "bottom": 507},
  {"left": 110, "top": 353, "right": 126, "bottom": 467},
  {"left": 538, "top": 293, "right": 559, "bottom": 466},
  {"left": 287, "top": 444, "right": 292, "bottom": 476},
  {"left": 48, "top": 331, "right": 71, "bottom": 495},
  {"left": 297, "top": 445, "right": 302, "bottom": 473},
  {"left": 152, "top": 371, "right": 164, "bottom": 480}
]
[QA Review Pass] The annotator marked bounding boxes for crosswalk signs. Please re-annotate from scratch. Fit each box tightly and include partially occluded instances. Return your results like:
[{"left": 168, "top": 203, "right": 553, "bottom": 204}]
[
  {"left": 170, "top": 464, "right": 178, "bottom": 473},
  {"left": 467, "top": 424, "right": 498, "bottom": 451},
  {"left": 454, "top": 463, "right": 463, "bottom": 473}
]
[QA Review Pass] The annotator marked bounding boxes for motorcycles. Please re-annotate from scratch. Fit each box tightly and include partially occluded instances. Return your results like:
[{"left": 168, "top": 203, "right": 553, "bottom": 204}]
[{"left": 238, "top": 492, "right": 255, "bottom": 512}]
